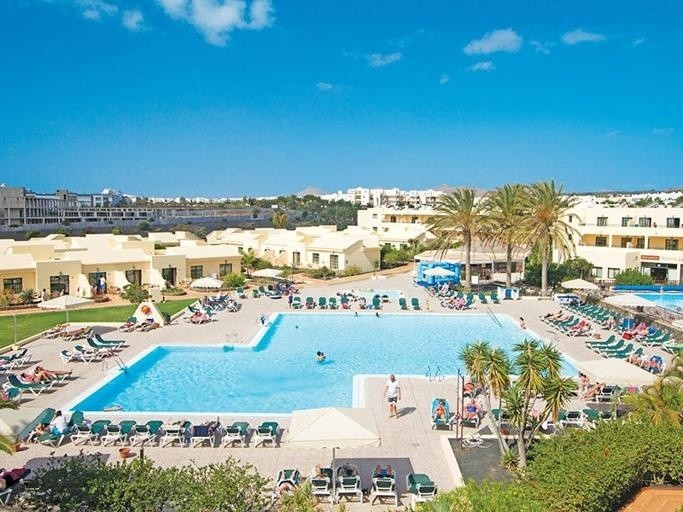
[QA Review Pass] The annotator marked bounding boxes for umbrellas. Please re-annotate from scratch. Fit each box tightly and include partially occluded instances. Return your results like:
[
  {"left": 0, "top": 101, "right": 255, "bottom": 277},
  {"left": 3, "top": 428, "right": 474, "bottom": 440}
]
[
  {"left": 189, "top": 277, "right": 224, "bottom": 305},
  {"left": 286, "top": 406, "right": 380, "bottom": 499},
  {"left": 603, "top": 291, "right": 656, "bottom": 330},
  {"left": 37, "top": 294, "right": 95, "bottom": 335},
  {"left": 559, "top": 278, "right": 598, "bottom": 308},
  {"left": 421, "top": 266, "right": 455, "bottom": 290},
  {"left": 250, "top": 267, "right": 283, "bottom": 291}
]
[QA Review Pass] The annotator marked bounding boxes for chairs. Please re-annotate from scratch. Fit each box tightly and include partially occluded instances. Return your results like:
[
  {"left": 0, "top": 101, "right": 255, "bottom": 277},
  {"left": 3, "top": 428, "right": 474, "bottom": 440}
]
[
  {"left": 0, "top": 332, "right": 126, "bottom": 406},
  {"left": 434, "top": 286, "right": 499, "bottom": 309},
  {"left": 540, "top": 299, "right": 683, "bottom": 372},
  {"left": 430, "top": 397, "right": 601, "bottom": 430},
  {"left": 187, "top": 281, "right": 281, "bottom": 316},
  {"left": 17, "top": 409, "right": 280, "bottom": 449},
  {"left": 271, "top": 463, "right": 438, "bottom": 508},
  {"left": 291, "top": 297, "right": 420, "bottom": 311}
]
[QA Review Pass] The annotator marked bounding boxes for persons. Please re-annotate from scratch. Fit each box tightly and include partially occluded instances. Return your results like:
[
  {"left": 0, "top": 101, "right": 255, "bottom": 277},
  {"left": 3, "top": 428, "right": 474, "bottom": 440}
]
[
  {"left": 81, "top": 345, "right": 113, "bottom": 355},
  {"left": 431, "top": 399, "right": 447, "bottom": 425},
  {"left": 201, "top": 416, "right": 221, "bottom": 436},
  {"left": 373, "top": 460, "right": 395, "bottom": 493},
  {"left": 336, "top": 461, "right": 362, "bottom": 489},
  {"left": 66, "top": 351, "right": 96, "bottom": 360},
  {"left": 463, "top": 382, "right": 474, "bottom": 391},
  {"left": 32, "top": 421, "right": 49, "bottom": 444},
  {"left": 577, "top": 369, "right": 590, "bottom": 393},
  {"left": 429, "top": 281, "right": 467, "bottom": 311},
  {"left": 287, "top": 291, "right": 292, "bottom": 309},
  {"left": 463, "top": 398, "right": 481, "bottom": 424},
  {"left": 309, "top": 461, "right": 331, "bottom": 484},
  {"left": 188, "top": 290, "right": 238, "bottom": 324},
  {"left": 519, "top": 315, "right": 526, "bottom": 330},
  {"left": 99, "top": 274, "right": 107, "bottom": 295},
  {"left": 44, "top": 410, "right": 69, "bottom": 436},
  {"left": 578, "top": 382, "right": 605, "bottom": 400},
  {"left": 272, "top": 463, "right": 299, "bottom": 498},
  {"left": 544, "top": 309, "right": 659, "bottom": 376},
  {"left": 40, "top": 288, "right": 47, "bottom": 302},
  {"left": 315, "top": 351, "right": 325, "bottom": 362},
  {"left": 168, "top": 416, "right": 186, "bottom": 439},
  {"left": 382, "top": 374, "right": 401, "bottom": 418},
  {"left": 0, "top": 466, "right": 28, "bottom": 491},
  {"left": 20, "top": 370, "right": 54, "bottom": 383},
  {"left": 35, "top": 366, "right": 70, "bottom": 376},
  {"left": 279, "top": 281, "right": 299, "bottom": 294}
]
